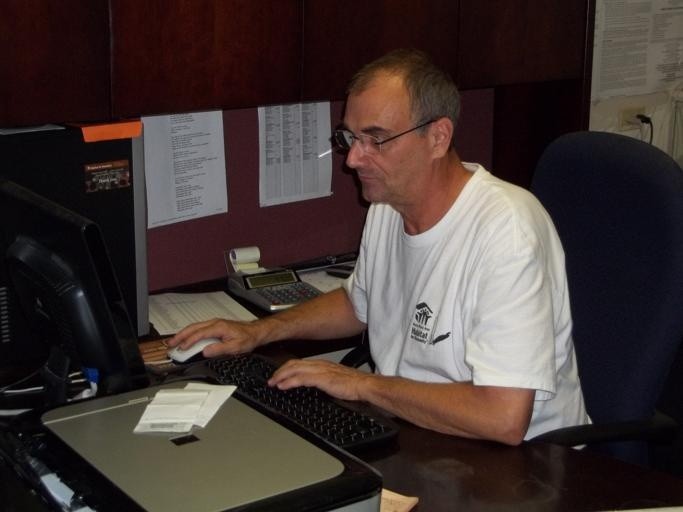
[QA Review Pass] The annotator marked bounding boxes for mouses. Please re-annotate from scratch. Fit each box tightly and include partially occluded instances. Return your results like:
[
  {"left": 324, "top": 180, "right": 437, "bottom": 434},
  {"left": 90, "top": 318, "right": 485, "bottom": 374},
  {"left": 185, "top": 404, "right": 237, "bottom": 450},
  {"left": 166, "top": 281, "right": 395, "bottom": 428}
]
[{"left": 167, "top": 337, "right": 222, "bottom": 364}]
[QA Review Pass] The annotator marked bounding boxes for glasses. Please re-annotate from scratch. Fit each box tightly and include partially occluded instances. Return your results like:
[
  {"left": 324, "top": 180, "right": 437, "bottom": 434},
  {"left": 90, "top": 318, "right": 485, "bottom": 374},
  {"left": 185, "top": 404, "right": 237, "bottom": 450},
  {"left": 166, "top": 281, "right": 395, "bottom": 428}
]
[{"left": 334, "top": 119, "right": 438, "bottom": 155}]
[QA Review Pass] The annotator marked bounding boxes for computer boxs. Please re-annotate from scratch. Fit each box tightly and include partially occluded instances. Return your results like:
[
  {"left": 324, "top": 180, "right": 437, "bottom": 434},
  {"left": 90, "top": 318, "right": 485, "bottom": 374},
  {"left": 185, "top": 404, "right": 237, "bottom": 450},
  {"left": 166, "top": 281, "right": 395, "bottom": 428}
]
[{"left": 0, "top": 116, "right": 151, "bottom": 338}]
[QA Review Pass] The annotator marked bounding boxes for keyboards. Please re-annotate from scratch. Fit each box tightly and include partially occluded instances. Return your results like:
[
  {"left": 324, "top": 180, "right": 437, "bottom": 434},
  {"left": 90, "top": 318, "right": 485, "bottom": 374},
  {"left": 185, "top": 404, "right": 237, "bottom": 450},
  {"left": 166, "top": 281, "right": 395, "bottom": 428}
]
[{"left": 184, "top": 351, "right": 401, "bottom": 448}]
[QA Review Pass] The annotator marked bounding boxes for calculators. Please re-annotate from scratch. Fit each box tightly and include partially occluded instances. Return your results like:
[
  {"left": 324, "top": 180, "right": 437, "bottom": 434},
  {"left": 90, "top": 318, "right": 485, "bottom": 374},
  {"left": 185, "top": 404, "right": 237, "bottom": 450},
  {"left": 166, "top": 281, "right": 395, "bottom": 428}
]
[{"left": 227, "top": 269, "right": 324, "bottom": 309}]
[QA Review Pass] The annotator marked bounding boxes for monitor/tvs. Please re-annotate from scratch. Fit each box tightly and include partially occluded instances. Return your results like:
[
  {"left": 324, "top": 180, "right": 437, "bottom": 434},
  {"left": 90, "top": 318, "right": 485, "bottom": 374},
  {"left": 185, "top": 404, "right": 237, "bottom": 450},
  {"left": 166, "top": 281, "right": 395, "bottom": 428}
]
[{"left": 0, "top": 180, "right": 151, "bottom": 436}]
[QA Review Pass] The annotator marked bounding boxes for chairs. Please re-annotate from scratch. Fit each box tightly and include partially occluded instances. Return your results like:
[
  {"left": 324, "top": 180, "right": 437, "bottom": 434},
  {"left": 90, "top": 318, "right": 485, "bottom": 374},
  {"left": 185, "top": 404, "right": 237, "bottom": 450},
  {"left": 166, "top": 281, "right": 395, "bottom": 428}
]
[{"left": 529, "top": 131, "right": 682, "bottom": 476}]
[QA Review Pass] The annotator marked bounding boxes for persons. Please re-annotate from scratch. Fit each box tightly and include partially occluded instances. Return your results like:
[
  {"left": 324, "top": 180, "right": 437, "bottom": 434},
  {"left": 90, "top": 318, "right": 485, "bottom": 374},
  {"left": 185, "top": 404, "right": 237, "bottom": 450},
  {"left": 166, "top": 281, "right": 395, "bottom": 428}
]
[{"left": 166, "top": 51, "right": 592, "bottom": 446}]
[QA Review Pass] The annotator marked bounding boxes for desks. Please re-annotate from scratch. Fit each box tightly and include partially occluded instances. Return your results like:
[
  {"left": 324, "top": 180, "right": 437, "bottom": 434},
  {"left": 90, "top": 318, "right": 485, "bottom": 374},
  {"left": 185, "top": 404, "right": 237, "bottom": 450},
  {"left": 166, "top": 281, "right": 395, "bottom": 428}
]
[{"left": 0, "top": 254, "right": 683, "bottom": 512}]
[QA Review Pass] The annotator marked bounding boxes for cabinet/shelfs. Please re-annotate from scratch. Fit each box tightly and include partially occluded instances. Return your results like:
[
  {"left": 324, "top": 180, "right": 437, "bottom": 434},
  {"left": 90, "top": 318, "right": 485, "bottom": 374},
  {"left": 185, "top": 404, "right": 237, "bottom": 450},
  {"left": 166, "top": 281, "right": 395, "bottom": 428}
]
[{"left": 1, "top": 0, "right": 595, "bottom": 130}]
